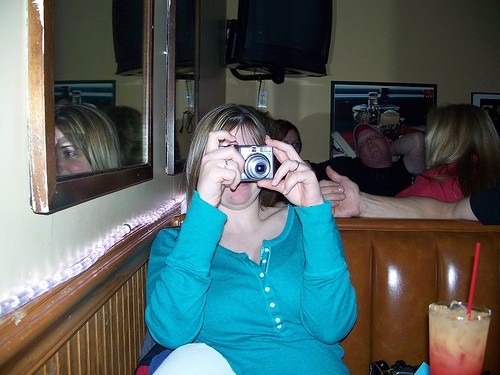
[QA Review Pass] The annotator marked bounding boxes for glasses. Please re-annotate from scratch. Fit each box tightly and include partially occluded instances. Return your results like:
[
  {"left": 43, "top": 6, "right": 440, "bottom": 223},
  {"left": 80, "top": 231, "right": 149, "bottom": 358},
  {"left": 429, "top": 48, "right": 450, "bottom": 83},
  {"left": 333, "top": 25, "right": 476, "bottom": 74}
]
[{"left": 286, "top": 142, "right": 302, "bottom": 148}]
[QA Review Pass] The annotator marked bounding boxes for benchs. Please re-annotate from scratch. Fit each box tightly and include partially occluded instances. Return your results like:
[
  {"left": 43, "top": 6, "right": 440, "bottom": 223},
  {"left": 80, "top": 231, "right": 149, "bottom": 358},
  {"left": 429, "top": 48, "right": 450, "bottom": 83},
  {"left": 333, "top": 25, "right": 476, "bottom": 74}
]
[{"left": 334, "top": 217, "right": 500, "bottom": 375}]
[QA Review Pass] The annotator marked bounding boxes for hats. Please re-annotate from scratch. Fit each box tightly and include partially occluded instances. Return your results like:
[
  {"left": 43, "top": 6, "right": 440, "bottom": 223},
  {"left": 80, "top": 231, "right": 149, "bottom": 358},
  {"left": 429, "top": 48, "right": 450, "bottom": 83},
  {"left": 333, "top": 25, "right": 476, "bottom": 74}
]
[{"left": 353, "top": 123, "right": 384, "bottom": 150}]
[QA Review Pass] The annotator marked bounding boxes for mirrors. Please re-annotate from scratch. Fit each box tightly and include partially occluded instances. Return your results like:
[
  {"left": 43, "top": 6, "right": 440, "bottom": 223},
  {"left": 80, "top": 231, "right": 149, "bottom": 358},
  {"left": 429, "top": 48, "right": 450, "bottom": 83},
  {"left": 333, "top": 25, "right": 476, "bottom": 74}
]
[
  {"left": 166, "top": 0, "right": 200, "bottom": 176},
  {"left": 29, "top": 0, "right": 155, "bottom": 214}
]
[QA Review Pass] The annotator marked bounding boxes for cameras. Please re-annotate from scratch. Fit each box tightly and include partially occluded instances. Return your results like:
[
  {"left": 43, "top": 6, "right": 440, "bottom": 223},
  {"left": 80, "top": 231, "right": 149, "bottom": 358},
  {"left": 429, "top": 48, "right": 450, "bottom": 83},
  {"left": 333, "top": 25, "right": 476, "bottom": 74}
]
[{"left": 220, "top": 144, "right": 273, "bottom": 181}]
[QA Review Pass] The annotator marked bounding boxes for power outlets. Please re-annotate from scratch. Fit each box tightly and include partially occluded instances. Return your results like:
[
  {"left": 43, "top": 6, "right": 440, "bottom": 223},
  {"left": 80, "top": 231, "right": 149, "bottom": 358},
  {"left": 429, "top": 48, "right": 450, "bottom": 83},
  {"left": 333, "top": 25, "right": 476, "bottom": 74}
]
[
  {"left": 183, "top": 91, "right": 194, "bottom": 107},
  {"left": 257, "top": 90, "right": 268, "bottom": 108}
]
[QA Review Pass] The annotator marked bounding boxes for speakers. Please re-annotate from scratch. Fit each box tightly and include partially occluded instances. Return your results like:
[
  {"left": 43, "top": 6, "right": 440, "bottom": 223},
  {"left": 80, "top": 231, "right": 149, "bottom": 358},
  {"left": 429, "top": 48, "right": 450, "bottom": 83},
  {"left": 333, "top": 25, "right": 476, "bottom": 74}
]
[
  {"left": 111, "top": 0, "right": 146, "bottom": 77},
  {"left": 174, "top": 0, "right": 198, "bottom": 78},
  {"left": 230, "top": 0, "right": 332, "bottom": 78}
]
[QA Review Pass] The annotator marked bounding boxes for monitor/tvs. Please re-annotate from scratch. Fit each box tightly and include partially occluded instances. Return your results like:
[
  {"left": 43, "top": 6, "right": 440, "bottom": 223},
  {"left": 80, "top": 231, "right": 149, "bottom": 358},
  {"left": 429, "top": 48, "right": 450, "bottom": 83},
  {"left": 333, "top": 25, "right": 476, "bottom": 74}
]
[
  {"left": 52, "top": 80, "right": 116, "bottom": 109},
  {"left": 330, "top": 81, "right": 439, "bottom": 160}
]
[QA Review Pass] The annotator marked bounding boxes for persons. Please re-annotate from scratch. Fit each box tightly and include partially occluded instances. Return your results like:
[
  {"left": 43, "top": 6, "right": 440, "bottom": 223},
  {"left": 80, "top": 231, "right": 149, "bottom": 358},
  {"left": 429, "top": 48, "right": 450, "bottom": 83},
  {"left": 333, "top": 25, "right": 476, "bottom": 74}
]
[
  {"left": 315, "top": 165, "right": 500, "bottom": 226},
  {"left": 272, "top": 103, "right": 500, "bottom": 204},
  {"left": 144, "top": 104, "right": 358, "bottom": 375},
  {"left": 54, "top": 102, "right": 142, "bottom": 176}
]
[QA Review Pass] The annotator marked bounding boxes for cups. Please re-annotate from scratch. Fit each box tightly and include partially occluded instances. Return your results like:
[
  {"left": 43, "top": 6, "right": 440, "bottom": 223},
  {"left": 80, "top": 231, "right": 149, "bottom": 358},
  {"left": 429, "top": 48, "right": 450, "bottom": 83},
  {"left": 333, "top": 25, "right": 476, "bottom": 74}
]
[{"left": 426, "top": 301, "right": 491, "bottom": 375}]
